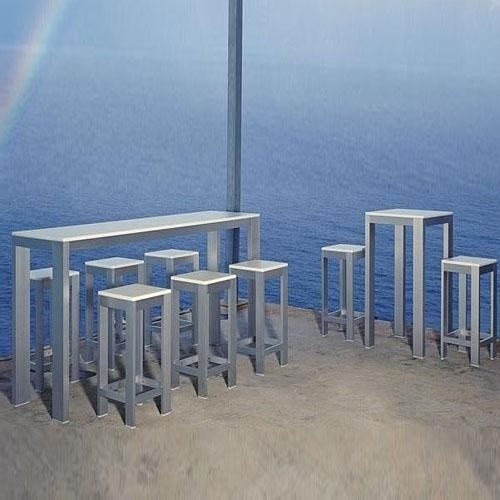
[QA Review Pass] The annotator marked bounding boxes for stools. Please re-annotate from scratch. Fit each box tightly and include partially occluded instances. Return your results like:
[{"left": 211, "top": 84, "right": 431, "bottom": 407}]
[
  {"left": 28, "top": 247, "right": 289, "bottom": 428},
  {"left": 320, "top": 242, "right": 365, "bottom": 343},
  {"left": 439, "top": 255, "right": 498, "bottom": 368}
]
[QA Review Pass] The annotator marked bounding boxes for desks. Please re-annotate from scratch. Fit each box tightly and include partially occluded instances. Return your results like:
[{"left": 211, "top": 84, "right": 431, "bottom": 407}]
[
  {"left": 12, "top": 210, "right": 262, "bottom": 424},
  {"left": 362, "top": 208, "right": 454, "bottom": 362}
]
